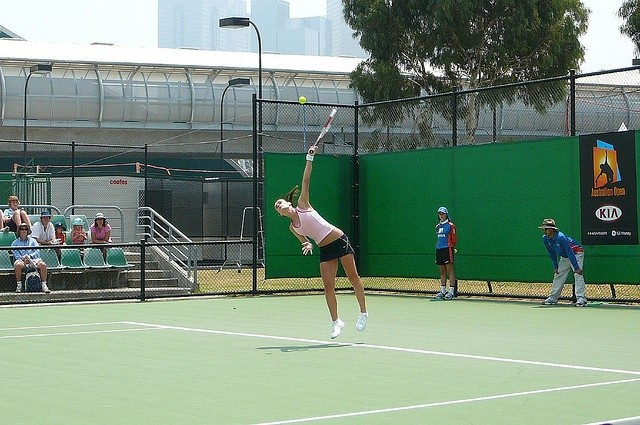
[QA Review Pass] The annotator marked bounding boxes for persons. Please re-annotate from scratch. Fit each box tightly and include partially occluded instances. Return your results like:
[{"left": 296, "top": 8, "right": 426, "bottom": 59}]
[
  {"left": 537, "top": 218, "right": 588, "bottom": 307},
  {"left": 0, "top": 209, "right": 3, "bottom": 232},
  {"left": 3, "top": 195, "right": 33, "bottom": 238},
  {"left": 70, "top": 217, "right": 87, "bottom": 245},
  {"left": 434, "top": 206, "right": 457, "bottom": 300},
  {"left": 273, "top": 146, "right": 368, "bottom": 339},
  {"left": 89, "top": 212, "right": 113, "bottom": 243},
  {"left": 53, "top": 221, "right": 65, "bottom": 258},
  {"left": 10, "top": 223, "right": 50, "bottom": 293},
  {"left": 31, "top": 211, "right": 55, "bottom": 245}
]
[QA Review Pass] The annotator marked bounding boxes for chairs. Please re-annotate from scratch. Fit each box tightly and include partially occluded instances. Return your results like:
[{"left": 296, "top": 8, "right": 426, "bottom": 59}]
[
  {"left": 39, "top": 249, "right": 68, "bottom": 289},
  {"left": 82, "top": 248, "right": 115, "bottom": 290},
  {"left": 0, "top": 250, "right": 15, "bottom": 274},
  {"left": 0, "top": 231, "right": 16, "bottom": 247},
  {"left": 53, "top": 215, "right": 69, "bottom": 230},
  {"left": 28, "top": 215, "right": 42, "bottom": 226},
  {"left": 105, "top": 247, "right": 135, "bottom": 289},
  {"left": 70, "top": 214, "right": 90, "bottom": 239},
  {"left": 60, "top": 249, "right": 90, "bottom": 290}
]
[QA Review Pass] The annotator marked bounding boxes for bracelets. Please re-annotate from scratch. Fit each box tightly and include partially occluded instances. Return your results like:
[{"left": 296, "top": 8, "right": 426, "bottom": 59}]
[{"left": 305, "top": 154, "right": 315, "bottom": 161}]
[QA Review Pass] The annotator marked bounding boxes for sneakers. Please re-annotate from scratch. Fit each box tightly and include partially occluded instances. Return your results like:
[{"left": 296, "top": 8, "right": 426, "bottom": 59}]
[
  {"left": 355, "top": 311, "right": 368, "bottom": 331},
  {"left": 542, "top": 299, "right": 556, "bottom": 305},
  {"left": 435, "top": 292, "right": 445, "bottom": 301},
  {"left": 444, "top": 292, "right": 454, "bottom": 300},
  {"left": 574, "top": 300, "right": 587, "bottom": 307},
  {"left": 331, "top": 318, "right": 345, "bottom": 339},
  {"left": 41, "top": 281, "right": 50, "bottom": 292},
  {"left": 15, "top": 281, "right": 22, "bottom": 292}
]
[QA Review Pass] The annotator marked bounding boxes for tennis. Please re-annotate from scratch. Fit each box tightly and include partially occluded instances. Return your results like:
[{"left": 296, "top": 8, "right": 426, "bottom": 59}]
[{"left": 298, "top": 97, "right": 307, "bottom": 105}]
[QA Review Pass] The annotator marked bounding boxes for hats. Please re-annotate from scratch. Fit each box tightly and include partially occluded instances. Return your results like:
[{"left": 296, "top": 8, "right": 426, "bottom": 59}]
[
  {"left": 40, "top": 211, "right": 51, "bottom": 220},
  {"left": 17, "top": 223, "right": 32, "bottom": 235},
  {"left": 72, "top": 218, "right": 85, "bottom": 226},
  {"left": 537, "top": 218, "right": 558, "bottom": 230},
  {"left": 437, "top": 206, "right": 448, "bottom": 214},
  {"left": 94, "top": 213, "right": 106, "bottom": 220},
  {"left": 54, "top": 222, "right": 67, "bottom": 231}
]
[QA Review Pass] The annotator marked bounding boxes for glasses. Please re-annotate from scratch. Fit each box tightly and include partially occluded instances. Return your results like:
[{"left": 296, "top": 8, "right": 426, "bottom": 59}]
[{"left": 20, "top": 228, "right": 28, "bottom": 230}]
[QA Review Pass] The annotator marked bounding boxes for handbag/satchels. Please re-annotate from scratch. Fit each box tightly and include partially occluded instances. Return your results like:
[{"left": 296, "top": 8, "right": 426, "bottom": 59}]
[{"left": 24, "top": 261, "right": 42, "bottom": 291}]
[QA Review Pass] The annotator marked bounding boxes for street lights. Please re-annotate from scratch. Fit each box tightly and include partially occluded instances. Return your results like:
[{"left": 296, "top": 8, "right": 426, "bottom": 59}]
[
  {"left": 221, "top": 78, "right": 250, "bottom": 237},
  {"left": 219, "top": 18, "right": 262, "bottom": 266},
  {"left": 24, "top": 64, "right": 52, "bottom": 160}
]
